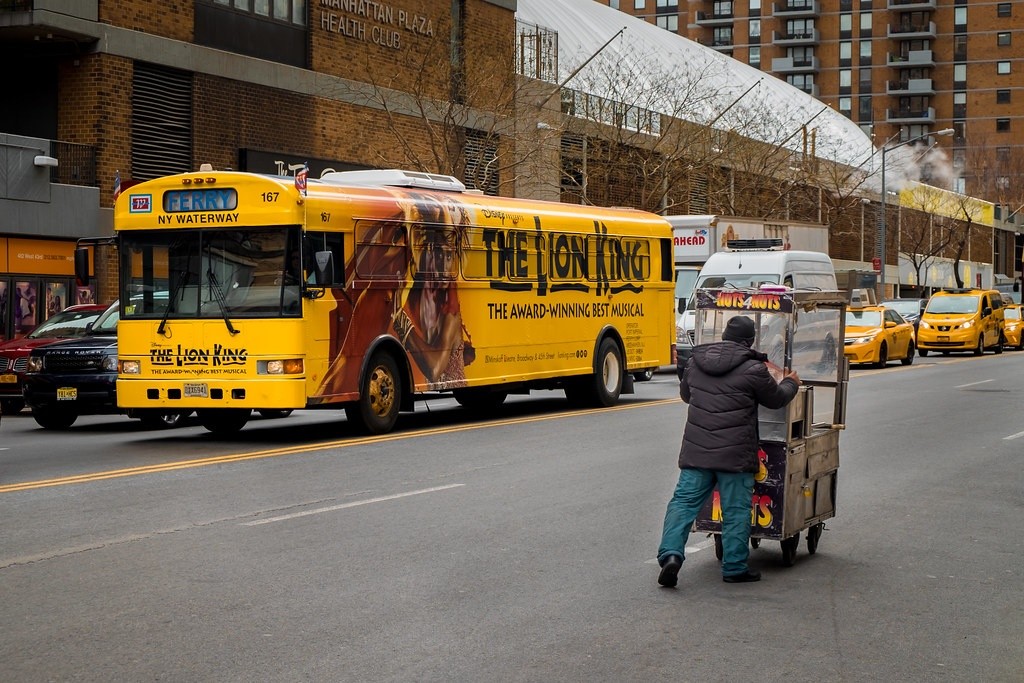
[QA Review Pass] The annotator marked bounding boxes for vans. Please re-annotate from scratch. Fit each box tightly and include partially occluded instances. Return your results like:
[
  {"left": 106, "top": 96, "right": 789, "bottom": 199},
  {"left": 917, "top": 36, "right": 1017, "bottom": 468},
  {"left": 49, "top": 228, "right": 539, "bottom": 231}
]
[
  {"left": 917, "top": 287, "right": 1007, "bottom": 359},
  {"left": 677, "top": 238, "right": 844, "bottom": 383}
]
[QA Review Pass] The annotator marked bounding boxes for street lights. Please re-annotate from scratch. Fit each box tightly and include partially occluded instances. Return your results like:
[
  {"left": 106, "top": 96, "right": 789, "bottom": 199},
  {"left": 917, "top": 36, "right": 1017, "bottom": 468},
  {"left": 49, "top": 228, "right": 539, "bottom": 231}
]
[
  {"left": 785, "top": 181, "right": 797, "bottom": 221},
  {"left": 712, "top": 148, "right": 736, "bottom": 216},
  {"left": 537, "top": 123, "right": 587, "bottom": 206},
  {"left": 789, "top": 166, "right": 823, "bottom": 223},
  {"left": 879, "top": 128, "right": 957, "bottom": 302},
  {"left": 661, "top": 154, "right": 677, "bottom": 215},
  {"left": 857, "top": 198, "right": 871, "bottom": 263}
]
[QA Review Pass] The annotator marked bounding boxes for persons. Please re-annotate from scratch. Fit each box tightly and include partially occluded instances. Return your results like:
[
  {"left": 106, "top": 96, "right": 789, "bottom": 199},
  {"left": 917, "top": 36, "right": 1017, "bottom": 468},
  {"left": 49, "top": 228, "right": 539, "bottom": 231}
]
[
  {"left": 391, "top": 194, "right": 467, "bottom": 388},
  {"left": 0, "top": 287, "right": 91, "bottom": 334},
  {"left": 656, "top": 316, "right": 802, "bottom": 586}
]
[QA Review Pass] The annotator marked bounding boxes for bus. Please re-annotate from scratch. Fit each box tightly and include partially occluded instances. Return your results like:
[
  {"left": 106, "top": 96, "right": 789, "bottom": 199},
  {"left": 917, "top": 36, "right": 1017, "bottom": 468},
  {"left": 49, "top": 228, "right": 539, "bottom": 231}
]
[{"left": 73, "top": 164, "right": 680, "bottom": 437}]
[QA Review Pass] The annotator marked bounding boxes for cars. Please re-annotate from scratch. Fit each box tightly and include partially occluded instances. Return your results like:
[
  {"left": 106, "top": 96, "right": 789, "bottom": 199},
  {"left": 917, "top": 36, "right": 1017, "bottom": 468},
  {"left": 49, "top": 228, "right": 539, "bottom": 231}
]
[
  {"left": 1000, "top": 303, "right": 1024, "bottom": 351},
  {"left": 837, "top": 287, "right": 916, "bottom": 370},
  {"left": 876, "top": 298, "right": 931, "bottom": 339}
]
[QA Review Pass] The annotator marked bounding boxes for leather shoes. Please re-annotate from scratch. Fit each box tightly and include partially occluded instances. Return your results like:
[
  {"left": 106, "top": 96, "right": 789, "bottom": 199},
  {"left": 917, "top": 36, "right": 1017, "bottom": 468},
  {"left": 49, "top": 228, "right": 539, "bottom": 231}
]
[
  {"left": 658, "top": 555, "right": 681, "bottom": 587},
  {"left": 722, "top": 571, "right": 761, "bottom": 582}
]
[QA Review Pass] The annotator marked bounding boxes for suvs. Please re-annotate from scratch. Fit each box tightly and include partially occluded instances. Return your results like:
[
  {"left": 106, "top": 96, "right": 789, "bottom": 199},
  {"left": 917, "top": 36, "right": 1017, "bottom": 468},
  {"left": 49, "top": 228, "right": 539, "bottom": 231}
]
[
  {"left": 0, "top": 301, "right": 124, "bottom": 416},
  {"left": 22, "top": 284, "right": 223, "bottom": 431}
]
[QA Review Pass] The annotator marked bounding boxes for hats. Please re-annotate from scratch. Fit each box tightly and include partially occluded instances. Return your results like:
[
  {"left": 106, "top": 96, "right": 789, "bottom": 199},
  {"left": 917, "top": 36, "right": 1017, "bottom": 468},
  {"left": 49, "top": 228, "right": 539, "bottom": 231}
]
[{"left": 722, "top": 315, "right": 756, "bottom": 344}]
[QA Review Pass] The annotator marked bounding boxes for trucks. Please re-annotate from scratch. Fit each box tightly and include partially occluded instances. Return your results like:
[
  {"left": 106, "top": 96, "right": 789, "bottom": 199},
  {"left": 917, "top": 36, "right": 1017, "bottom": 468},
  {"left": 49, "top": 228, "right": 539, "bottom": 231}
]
[{"left": 659, "top": 214, "right": 831, "bottom": 319}]
